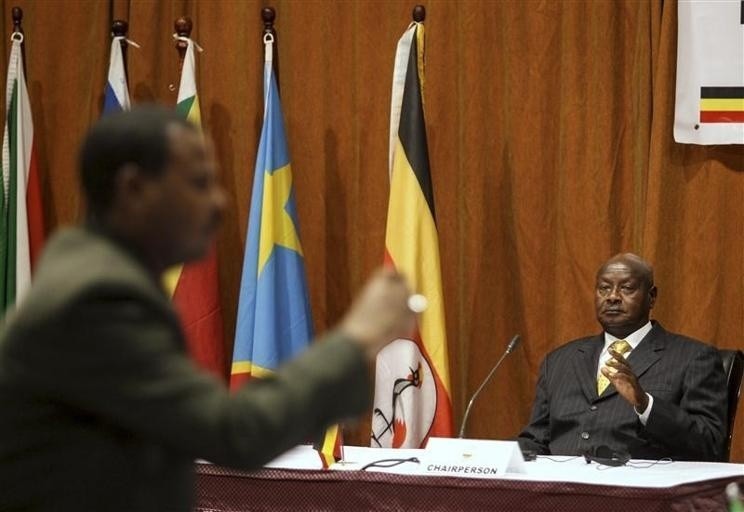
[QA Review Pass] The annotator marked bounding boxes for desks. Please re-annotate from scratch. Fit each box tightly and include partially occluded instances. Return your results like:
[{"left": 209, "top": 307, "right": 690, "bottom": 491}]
[{"left": 188, "top": 441, "right": 743, "bottom": 512}]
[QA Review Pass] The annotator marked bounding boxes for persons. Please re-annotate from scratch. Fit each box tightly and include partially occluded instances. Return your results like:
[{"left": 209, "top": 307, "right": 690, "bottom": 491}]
[
  {"left": 0, "top": 106, "right": 415, "bottom": 510},
  {"left": 513, "top": 253, "right": 734, "bottom": 466}
]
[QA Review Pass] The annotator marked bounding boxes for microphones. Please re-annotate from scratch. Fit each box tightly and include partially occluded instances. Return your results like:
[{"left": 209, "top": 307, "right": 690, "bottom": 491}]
[{"left": 456, "top": 333, "right": 521, "bottom": 437}]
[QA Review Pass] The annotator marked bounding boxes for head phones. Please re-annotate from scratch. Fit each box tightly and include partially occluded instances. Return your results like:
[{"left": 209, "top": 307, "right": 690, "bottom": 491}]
[{"left": 580, "top": 442, "right": 632, "bottom": 467}]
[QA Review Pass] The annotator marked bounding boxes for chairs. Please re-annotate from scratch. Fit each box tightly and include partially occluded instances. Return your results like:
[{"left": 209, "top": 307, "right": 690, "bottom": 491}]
[{"left": 708, "top": 350, "right": 742, "bottom": 463}]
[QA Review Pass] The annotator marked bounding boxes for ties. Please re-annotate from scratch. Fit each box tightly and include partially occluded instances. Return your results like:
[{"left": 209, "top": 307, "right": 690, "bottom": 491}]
[{"left": 597, "top": 341, "right": 632, "bottom": 397}]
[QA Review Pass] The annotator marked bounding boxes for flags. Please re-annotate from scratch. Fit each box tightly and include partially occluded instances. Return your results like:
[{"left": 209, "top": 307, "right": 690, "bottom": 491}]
[
  {"left": 162, "top": 39, "right": 225, "bottom": 388},
  {"left": 370, "top": 20, "right": 458, "bottom": 448},
  {"left": 224, "top": 30, "right": 348, "bottom": 471},
  {"left": 92, "top": 34, "right": 140, "bottom": 118},
  {"left": 0, "top": 35, "right": 54, "bottom": 350}
]
